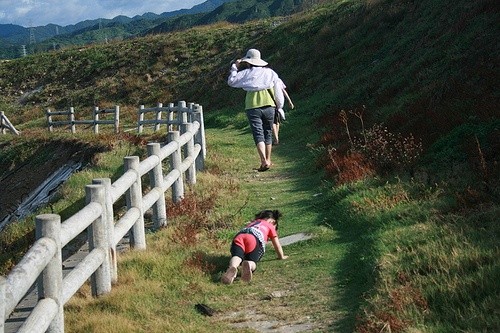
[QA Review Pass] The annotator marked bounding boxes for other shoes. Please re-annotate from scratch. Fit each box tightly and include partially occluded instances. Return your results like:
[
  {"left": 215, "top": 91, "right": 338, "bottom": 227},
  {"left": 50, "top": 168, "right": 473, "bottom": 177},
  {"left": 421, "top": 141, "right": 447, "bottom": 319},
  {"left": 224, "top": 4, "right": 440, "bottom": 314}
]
[
  {"left": 241, "top": 261, "right": 252, "bottom": 281},
  {"left": 222, "top": 266, "right": 236, "bottom": 285}
]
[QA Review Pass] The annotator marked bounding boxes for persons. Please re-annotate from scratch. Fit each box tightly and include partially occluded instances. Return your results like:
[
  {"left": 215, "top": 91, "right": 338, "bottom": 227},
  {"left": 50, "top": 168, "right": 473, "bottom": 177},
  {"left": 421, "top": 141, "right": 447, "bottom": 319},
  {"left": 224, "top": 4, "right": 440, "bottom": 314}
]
[
  {"left": 228, "top": 49, "right": 284, "bottom": 172},
  {"left": 222, "top": 210, "right": 289, "bottom": 284},
  {"left": 273, "top": 77, "right": 294, "bottom": 144}
]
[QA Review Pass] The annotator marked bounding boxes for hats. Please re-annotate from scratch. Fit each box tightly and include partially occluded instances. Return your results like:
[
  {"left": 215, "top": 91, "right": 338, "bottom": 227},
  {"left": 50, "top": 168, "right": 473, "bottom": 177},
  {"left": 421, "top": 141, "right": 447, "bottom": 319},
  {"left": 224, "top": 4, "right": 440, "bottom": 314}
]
[{"left": 238, "top": 49, "right": 268, "bottom": 66}]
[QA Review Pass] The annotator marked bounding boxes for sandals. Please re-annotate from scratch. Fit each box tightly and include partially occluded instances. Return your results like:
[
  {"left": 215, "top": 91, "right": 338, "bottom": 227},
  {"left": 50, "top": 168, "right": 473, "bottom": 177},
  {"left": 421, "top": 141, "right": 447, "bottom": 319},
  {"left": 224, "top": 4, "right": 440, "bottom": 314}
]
[{"left": 258, "top": 164, "right": 269, "bottom": 172}]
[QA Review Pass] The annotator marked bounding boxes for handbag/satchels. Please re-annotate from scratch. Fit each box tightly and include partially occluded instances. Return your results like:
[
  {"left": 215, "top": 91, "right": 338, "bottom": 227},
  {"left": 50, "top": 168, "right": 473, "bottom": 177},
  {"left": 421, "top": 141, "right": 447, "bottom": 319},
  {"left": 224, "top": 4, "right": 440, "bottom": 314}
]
[{"left": 274, "top": 108, "right": 280, "bottom": 125}]
